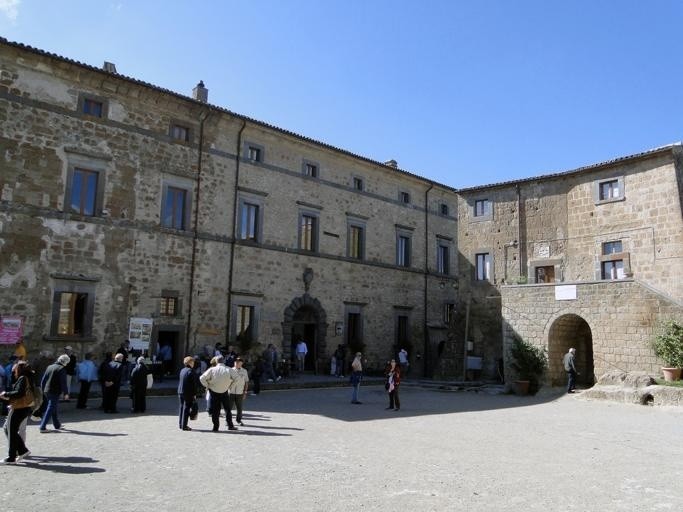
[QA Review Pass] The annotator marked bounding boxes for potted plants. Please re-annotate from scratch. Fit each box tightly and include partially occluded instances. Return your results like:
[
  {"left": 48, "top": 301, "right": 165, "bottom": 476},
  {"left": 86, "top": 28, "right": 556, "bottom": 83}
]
[
  {"left": 651, "top": 319, "right": 683, "bottom": 381},
  {"left": 510, "top": 337, "right": 546, "bottom": 396}
]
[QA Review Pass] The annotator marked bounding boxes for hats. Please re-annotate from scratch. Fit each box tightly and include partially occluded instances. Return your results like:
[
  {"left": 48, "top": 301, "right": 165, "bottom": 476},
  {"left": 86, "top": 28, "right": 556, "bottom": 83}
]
[
  {"left": 235, "top": 356, "right": 244, "bottom": 363},
  {"left": 12, "top": 345, "right": 25, "bottom": 356},
  {"left": 210, "top": 356, "right": 216, "bottom": 364},
  {"left": 64, "top": 345, "right": 73, "bottom": 351},
  {"left": 183, "top": 356, "right": 196, "bottom": 364}
]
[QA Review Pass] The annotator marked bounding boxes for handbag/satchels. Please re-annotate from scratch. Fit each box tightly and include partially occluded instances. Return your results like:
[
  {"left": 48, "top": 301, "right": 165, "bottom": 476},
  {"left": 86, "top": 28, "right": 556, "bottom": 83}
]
[
  {"left": 146, "top": 373, "right": 154, "bottom": 389},
  {"left": 205, "top": 390, "right": 211, "bottom": 410},
  {"left": 10, "top": 375, "right": 35, "bottom": 408},
  {"left": 189, "top": 402, "right": 199, "bottom": 420},
  {"left": 385, "top": 382, "right": 390, "bottom": 391}
]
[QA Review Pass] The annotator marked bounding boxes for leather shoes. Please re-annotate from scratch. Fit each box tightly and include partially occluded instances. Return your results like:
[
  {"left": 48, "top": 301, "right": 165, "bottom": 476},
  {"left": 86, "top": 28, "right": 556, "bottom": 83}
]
[
  {"left": 235, "top": 419, "right": 243, "bottom": 426},
  {"left": 392, "top": 408, "right": 400, "bottom": 411},
  {"left": 385, "top": 407, "right": 394, "bottom": 410}
]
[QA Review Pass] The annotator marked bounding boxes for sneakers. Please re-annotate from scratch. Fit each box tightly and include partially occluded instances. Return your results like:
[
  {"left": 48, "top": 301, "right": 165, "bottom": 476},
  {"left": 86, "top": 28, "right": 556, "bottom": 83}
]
[
  {"left": 226, "top": 423, "right": 237, "bottom": 430},
  {"left": 77, "top": 405, "right": 93, "bottom": 410},
  {"left": 250, "top": 393, "right": 257, "bottom": 396},
  {"left": 567, "top": 385, "right": 577, "bottom": 393},
  {"left": 0, "top": 457, "right": 17, "bottom": 465},
  {"left": 16, "top": 449, "right": 31, "bottom": 462},
  {"left": 351, "top": 400, "right": 363, "bottom": 404},
  {"left": 30, "top": 415, "right": 42, "bottom": 422},
  {"left": 267, "top": 378, "right": 273, "bottom": 382},
  {"left": 276, "top": 375, "right": 281, "bottom": 382},
  {"left": 39, "top": 428, "right": 51, "bottom": 433},
  {"left": 55, "top": 424, "right": 67, "bottom": 430},
  {"left": 179, "top": 425, "right": 191, "bottom": 431},
  {"left": 212, "top": 425, "right": 219, "bottom": 432}
]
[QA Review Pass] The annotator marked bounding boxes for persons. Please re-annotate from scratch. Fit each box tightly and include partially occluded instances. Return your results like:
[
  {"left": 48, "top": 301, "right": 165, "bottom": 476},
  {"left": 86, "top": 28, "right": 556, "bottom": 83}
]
[
  {"left": 295, "top": 336, "right": 308, "bottom": 372},
  {"left": 562, "top": 347, "right": 582, "bottom": 394},
  {"left": 398, "top": 349, "right": 408, "bottom": 380},
  {"left": 0, "top": 340, "right": 281, "bottom": 465},
  {"left": 334, "top": 342, "right": 346, "bottom": 378},
  {"left": 350, "top": 351, "right": 364, "bottom": 405},
  {"left": 383, "top": 357, "right": 402, "bottom": 411}
]
